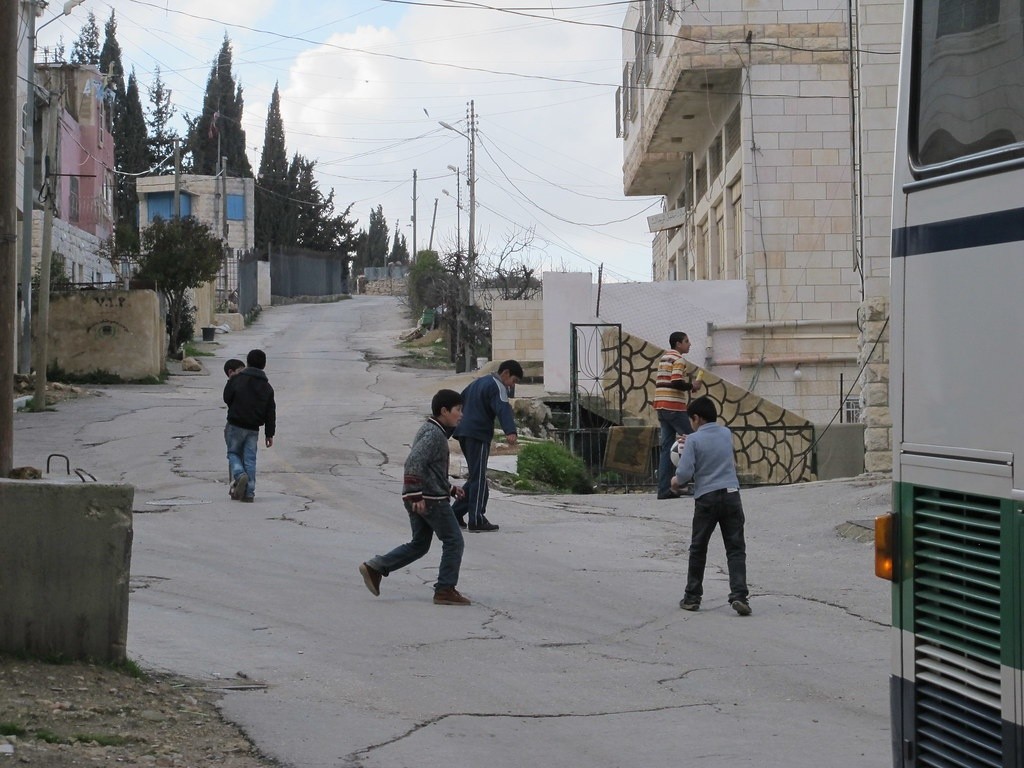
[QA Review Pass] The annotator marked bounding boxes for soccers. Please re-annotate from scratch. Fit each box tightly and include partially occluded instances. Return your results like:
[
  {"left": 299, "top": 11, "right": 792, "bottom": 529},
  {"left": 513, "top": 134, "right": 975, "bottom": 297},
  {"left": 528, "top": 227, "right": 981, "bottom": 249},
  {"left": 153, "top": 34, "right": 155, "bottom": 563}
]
[{"left": 668, "top": 437, "right": 689, "bottom": 469}]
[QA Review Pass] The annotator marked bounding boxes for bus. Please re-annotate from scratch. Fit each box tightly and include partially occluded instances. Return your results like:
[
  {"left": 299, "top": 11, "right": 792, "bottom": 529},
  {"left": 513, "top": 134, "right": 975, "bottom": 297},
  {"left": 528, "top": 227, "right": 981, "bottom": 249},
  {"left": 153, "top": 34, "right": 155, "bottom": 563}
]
[{"left": 876, "top": 0, "right": 1024, "bottom": 768}]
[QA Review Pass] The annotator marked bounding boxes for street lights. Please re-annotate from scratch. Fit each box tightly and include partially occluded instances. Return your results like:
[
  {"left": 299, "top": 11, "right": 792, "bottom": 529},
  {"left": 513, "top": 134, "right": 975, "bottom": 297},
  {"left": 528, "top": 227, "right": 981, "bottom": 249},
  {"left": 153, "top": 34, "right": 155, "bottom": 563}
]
[
  {"left": 439, "top": 121, "right": 475, "bottom": 306},
  {"left": 22, "top": 0, "right": 85, "bottom": 375}
]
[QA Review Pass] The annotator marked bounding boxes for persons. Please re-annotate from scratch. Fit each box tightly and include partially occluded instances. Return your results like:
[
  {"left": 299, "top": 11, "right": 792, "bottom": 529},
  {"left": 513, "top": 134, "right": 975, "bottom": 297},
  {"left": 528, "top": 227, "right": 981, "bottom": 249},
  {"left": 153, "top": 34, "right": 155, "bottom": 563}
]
[
  {"left": 671, "top": 396, "right": 751, "bottom": 615},
  {"left": 654, "top": 332, "right": 704, "bottom": 499},
  {"left": 451, "top": 360, "right": 523, "bottom": 533},
  {"left": 359, "top": 389, "right": 473, "bottom": 605},
  {"left": 223, "top": 349, "right": 276, "bottom": 501}
]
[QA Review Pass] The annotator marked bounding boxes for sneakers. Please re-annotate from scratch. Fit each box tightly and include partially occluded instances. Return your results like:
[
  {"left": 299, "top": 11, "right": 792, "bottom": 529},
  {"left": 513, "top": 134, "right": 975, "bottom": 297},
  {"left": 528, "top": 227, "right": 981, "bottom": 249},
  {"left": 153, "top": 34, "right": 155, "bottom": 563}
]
[
  {"left": 359, "top": 562, "right": 382, "bottom": 596},
  {"left": 680, "top": 599, "right": 699, "bottom": 611},
  {"left": 434, "top": 584, "right": 471, "bottom": 605},
  {"left": 453, "top": 511, "right": 467, "bottom": 528},
  {"left": 730, "top": 597, "right": 752, "bottom": 615},
  {"left": 469, "top": 521, "right": 499, "bottom": 533}
]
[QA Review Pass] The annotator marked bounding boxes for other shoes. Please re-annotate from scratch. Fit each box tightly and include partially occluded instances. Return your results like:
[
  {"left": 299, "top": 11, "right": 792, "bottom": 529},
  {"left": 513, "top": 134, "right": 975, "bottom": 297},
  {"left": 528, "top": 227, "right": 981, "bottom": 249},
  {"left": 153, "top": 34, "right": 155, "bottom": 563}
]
[
  {"left": 681, "top": 486, "right": 694, "bottom": 495},
  {"left": 242, "top": 493, "right": 253, "bottom": 502},
  {"left": 229, "top": 487, "right": 235, "bottom": 500},
  {"left": 233, "top": 474, "right": 248, "bottom": 499},
  {"left": 658, "top": 488, "right": 680, "bottom": 499}
]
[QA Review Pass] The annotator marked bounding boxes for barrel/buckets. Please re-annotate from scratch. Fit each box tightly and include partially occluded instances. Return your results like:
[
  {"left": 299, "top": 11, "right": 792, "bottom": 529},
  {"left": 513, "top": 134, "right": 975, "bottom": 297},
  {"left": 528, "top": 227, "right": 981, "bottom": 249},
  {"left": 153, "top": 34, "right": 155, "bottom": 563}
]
[{"left": 201, "top": 327, "right": 216, "bottom": 341}]
